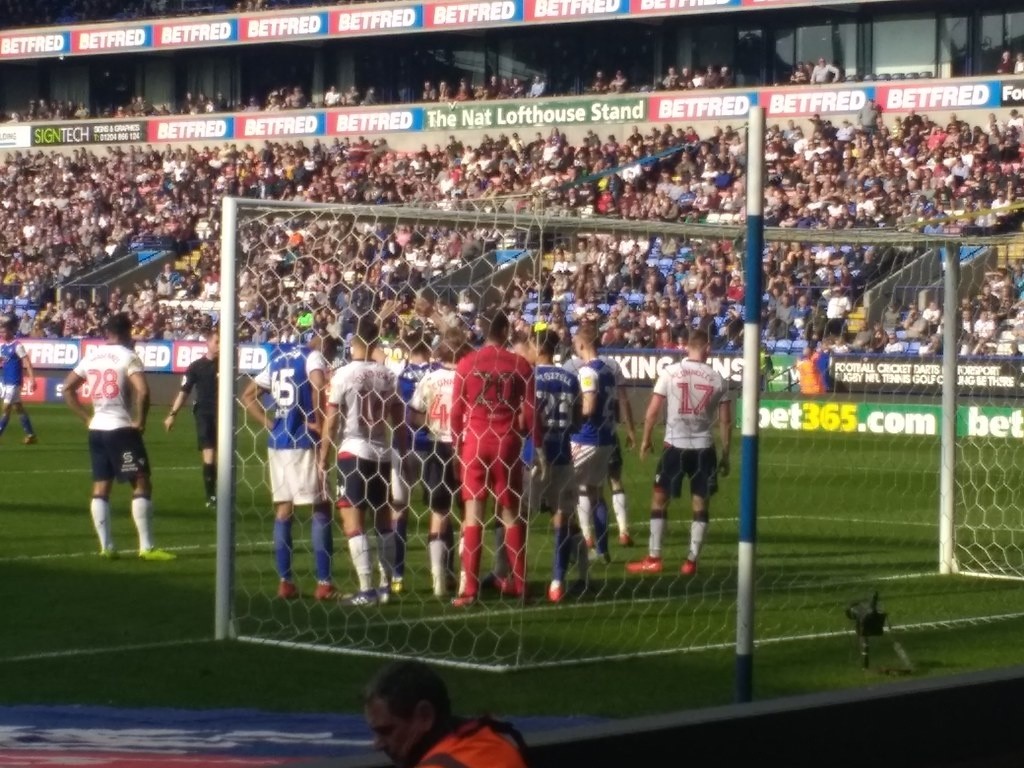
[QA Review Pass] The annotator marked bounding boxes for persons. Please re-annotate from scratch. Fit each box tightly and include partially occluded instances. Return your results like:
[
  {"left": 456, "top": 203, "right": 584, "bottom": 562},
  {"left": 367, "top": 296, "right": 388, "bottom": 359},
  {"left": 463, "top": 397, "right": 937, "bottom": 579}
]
[
  {"left": 364, "top": 658, "right": 535, "bottom": 768},
  {"left": 0, "top": 323, "right": 37, "bottom": 445},
  {"left": 62, "top": 311, "right": 178, "bottom": 561},
  {"left": 0, "top": 48, "right": 1024, "bottom": 359},
  {"left": 165, "top": 332, "right": 221, "bottom": 508},
  {"left": 626, "top": 330, "right": 732, "bottom": 573},
  {"left": 242, "top": 310, "right": 634, "bottom": 605}
]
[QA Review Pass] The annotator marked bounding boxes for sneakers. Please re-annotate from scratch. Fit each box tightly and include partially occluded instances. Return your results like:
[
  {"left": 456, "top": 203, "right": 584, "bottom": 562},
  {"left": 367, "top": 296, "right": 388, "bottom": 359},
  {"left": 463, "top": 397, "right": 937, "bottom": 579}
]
[
  {"left": 492, "top": 576, "right": 530, "bottom": 598},
  {"left": 626, "top": 555, "right": 663, "bottom": 574},
  {"left": 618, "top": 533, "right": 631, "bottom": 546},
  {"left": 680, "top": 559, "right": 696, "bottom": 576},
  {"left": 345, "top": 588, "right": 380, "bottom": 608},
  {"left": 278, "top": 582, "right": 297, "bottom": 598},
  {"left": 138, "top": 545, "right": 178, "bottom": 562},
  {"left": 546, "top": 580, "right": 565, "bottom": 603},
  {"left": 376, "top": 585, "right": 391, "bottom": 605},
  {"left": 99, "top": 549, "right": 120, "bottom": 561},
  {"left": 315, "top": 584, "right": 338, "bottom": 601}
]
